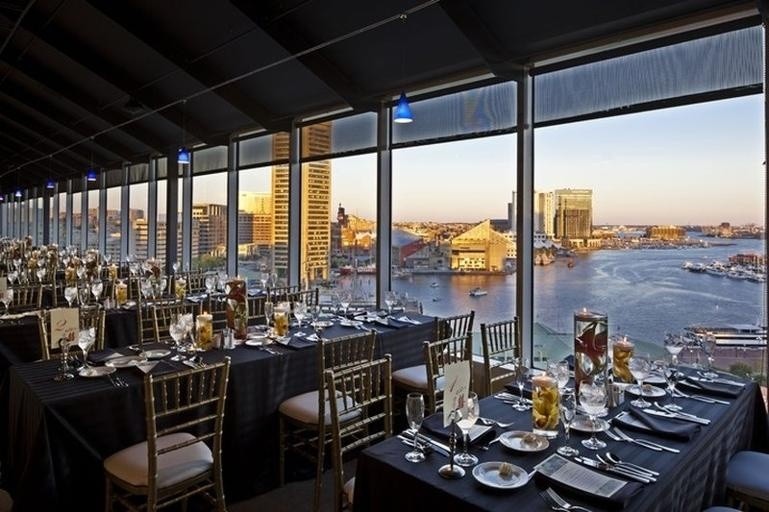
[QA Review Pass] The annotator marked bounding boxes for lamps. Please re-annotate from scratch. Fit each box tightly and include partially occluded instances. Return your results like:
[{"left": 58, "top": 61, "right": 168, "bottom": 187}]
[
  {"left": 390, "top": 11, "right": 416, "bottom": 126},
  {"left": 176, "top": 95, "right": 192, "bottom": 166},
  {"left": 85, "top": 137, "right": 100, "bottom": 184},
  {"left": 44, "top": 152, "right": 57, "bottom": 191}
]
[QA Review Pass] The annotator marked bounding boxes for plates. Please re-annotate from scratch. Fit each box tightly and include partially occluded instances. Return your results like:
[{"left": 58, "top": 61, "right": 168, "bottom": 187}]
[
  {"left": 80, "top": 346, "right": 171, "bottom": 380},
  {"left": 498, "top": 430, "right": 550, "bottom": 454},
  {"left": 471, "top": 461, "right": 532, "bottom": 489}
]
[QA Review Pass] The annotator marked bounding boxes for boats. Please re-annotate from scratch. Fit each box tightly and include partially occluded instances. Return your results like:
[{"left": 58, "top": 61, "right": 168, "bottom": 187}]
[
  {"left": 392, "top": 272, "right": 412, "bottom": 280},
  {"left": 567, "top": 257, "right": 577, "bottom": 268},
  {"left": 469, "top": 287, "right": 487, "bottom": 297},
  {"left": 665, "top": 321, "right": 767, "bottom": 354},
  {"left": 433, "top": 296, "right": 441, "bottom": 302},
  {"left": 430, "top": 283, "right": 439, "bottom": 288}
]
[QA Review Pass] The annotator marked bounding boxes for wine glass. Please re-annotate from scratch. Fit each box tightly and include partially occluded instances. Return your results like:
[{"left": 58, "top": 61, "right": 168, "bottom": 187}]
[
  {"left": 0, "top": 233, "right": 267, "bottom": 308},
  {"left": 264, "top": 290, "right": 422, "bottom": 351},
  {"left": 404, "top": 389, "right": 428, "bottom": 463},
  {"left": 75, "top": 325, "right": 95, "bottom": 376},
  {"left": 56, "top": 328, "right": 72, "bottom": 382},
  {"left": 510, "top": 305, "right": 717, "bottom": 457},
  {"left": 167, "top": 305, "right": 284, "bottom": 369},
  {"left": 455, "top": 390, "right": 481, "bottom": 468}
]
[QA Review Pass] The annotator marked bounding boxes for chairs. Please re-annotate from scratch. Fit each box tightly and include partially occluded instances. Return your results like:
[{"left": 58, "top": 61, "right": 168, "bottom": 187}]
[
  {"left": 279, "top": 327, "right": 377, "bottom": 508},
  {"left": 721, "top": 450, "right": 769, "bottom": 508},
  {"left": 392, "top": 310, "right": 474, "bottom": 415},
  {"left": 421, "top": 331, "right": 474, "bottom": 419},
  {"left": 480, "top": 316, "right": 523, "bottom": 396},
  {"left": 0, "top": 235, "right": 319, "bottom": 509},
  {"left": 325, "top": 354, "right": 392, "bottom": 508}
]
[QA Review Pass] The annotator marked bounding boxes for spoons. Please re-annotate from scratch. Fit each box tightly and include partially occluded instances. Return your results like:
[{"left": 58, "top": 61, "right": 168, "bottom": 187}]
[{"left": 573, "top": 451, "right": 661, "bottom": 485}]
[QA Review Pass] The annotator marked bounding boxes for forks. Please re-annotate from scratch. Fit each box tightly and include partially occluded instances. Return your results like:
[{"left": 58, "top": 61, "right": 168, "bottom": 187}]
[
  {"left": 538, "top": 486, "right": 592, "bottom": 511},
  {"left": 604, "top": 426, "right": 681, "bottom": 457},
  {"left": 662, "top": 364, "right": 746, "bottom": 408}
]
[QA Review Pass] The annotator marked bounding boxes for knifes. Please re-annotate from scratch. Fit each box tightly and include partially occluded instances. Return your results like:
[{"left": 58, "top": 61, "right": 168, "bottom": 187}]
[{"left": 398, "top": 426, "right": 450, "bottom": 455}]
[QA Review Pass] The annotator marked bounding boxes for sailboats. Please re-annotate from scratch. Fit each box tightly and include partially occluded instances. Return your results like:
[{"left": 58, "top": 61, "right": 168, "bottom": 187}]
[
  {"left": 533, "top": 249, "right": 556, "bottom": 266},
  {"left": 682, "top": 258, "right": 766, "bottom": 285}
]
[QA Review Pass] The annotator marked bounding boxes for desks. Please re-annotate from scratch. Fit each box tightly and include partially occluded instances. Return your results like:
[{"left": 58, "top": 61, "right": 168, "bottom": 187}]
[
  {"left": 4, "top": 309, "right": 452, "bottom": 508},
  {"left": 352, "top": 353, "right": 769, "bottom": 509}
]
